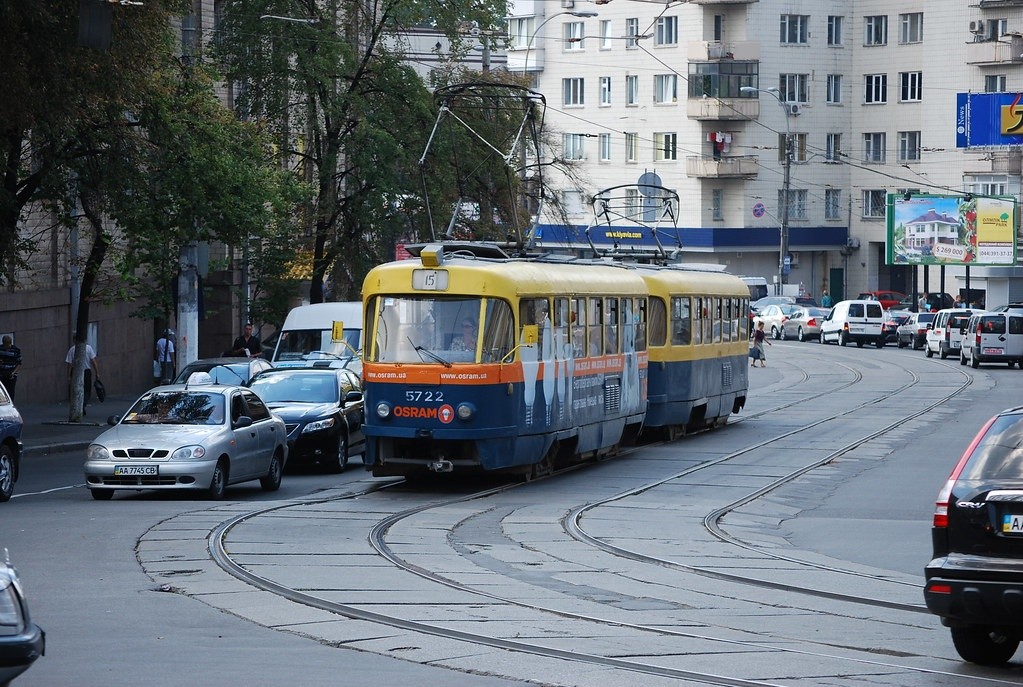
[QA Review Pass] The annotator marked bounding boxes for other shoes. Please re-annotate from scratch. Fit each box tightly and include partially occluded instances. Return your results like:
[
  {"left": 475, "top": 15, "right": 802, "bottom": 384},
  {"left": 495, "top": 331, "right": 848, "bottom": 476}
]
[
  {"left": 82, "top": 409, "right": 86, "bottom": 416},
  {"left": 761, "top": 364, "right": 766, "bottom": 367},
  {"left": 751, "top": 364, "right": 757, "bottom": 368}
]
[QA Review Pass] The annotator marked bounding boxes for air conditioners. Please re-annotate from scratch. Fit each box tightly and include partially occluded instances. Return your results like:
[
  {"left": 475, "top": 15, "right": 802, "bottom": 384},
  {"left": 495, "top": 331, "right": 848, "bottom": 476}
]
[
  {"left": 969, "top": 20, "right": 983, "bottom": 35},
  {"left": 790, "top": 103, "right": 802, "bottom": 117},
  {"left": 847, "top": 238, "right": 860, "bottom": 251},
  {"left": 787, "top": 252, "right": 798, "bottom": 264},
  {"left": 560, "top": 0, "right": 574, "bottom": 9}
]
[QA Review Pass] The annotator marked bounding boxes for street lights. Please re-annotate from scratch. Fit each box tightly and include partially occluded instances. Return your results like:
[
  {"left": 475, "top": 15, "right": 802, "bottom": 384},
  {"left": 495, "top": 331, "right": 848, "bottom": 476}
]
[
  {"left": 740, "top": 85, "right": 791, "bottom": 296},
  {"left": 518, "top": 10, "right": 601, "bottom": 210}
]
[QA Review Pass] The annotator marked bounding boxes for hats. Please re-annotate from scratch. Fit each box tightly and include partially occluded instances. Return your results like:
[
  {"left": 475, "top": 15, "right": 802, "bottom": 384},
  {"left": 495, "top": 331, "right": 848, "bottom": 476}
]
[{"left": 163, "top": 329, "right": 175, "bottom": 335}]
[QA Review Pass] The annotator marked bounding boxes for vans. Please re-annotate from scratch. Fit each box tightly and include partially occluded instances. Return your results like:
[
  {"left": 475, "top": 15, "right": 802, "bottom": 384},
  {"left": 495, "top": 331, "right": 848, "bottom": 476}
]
[
  {"left": 739, "top": 277, "right": 769, "bottom": 307},
  {"left": 819, "top": 300, "right": 885, "bottom": 349},
  {"left": 925, "top": 308, "right": 988, "bottom": 359},
  {"left": 959, "top": 312, "right": 1023, "bottom": 370},
  {"left": 269, "top": 298, "right": 407, "bottom": 380}
]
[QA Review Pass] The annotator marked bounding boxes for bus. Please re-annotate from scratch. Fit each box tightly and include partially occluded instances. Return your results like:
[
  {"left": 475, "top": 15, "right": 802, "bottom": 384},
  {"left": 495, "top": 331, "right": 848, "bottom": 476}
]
[{"left": 329, "top": 182, "right": 750, "bottom": 497}]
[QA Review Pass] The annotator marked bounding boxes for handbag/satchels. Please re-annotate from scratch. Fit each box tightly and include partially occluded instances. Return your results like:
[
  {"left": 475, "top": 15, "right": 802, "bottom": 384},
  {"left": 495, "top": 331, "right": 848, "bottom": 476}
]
[
  {"left": 749, "top": 347, "right": 760, "bottom": 359},
  {"left": 93, "top": 377, "right": 106, "bottom": 403},
  {"left": 153, "top": 356, "right": 162, "bottom": 378}
]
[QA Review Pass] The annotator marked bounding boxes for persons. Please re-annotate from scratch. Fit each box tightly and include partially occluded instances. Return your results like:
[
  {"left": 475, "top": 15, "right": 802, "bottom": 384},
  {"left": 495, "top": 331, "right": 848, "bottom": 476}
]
[
  {"left": 130, "top": 399, "right": 180, "bottom": 422},
  {"left": 751, "top": 321, "right": 772, "bottom": 368},
  {"left": 233, "top": 323, "right": 262, "bottom": 358},
  {"left": 448, "top": 317, "right": 486, "bottom": 353},
  {"left": 156, "top": 328, "right": 175, "bottom": 379},
  {"left": 0, "top": 335, "right": 23, "bottom": 404},
  {"left": 969, "top": 300, "right": 976, "bottom": 309},
  {"left": 821, "top": 290, "right": 833, "bottom": 308},
  {"left": 918, "top": 292, "right": 928, "bottom": 312},
  {"left": 65, "top": 332, "right": 99, "bottom": 415},
  {"left": 953, "top": 295, "right": 962, "bottom": 309}
]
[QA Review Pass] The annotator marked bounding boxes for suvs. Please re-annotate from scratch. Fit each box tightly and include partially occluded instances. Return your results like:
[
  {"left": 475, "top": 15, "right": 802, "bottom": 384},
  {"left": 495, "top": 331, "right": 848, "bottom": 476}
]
[
  {"left": 923, "top": 405, "right": 1023, "bottom": 668},
  {"left": 989, "top": 302, "right": 1023, "bottom": 315},
  {"left": 887, "top": 292, "right": 955, "bottom": 314}
]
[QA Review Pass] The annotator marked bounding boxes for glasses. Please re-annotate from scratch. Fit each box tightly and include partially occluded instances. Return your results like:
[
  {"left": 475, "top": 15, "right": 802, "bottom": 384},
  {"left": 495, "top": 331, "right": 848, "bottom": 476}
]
[{"left": 461, "top": 323, "right": 474, "bottom": 329}]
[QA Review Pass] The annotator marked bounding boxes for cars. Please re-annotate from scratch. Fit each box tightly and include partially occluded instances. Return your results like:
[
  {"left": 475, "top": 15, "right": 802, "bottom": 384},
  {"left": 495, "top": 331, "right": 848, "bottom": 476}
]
[
  {"left": 857, "top": 290, "right": 907, "bottom": 310},
  {"left": 883, "top": 310, "right": 913, "bottom": 345},
  {"left": 81, "top": 384, "right": 288, "bottom": 499},
  {"left": 750, "top": 295, "right": 831, "bottom": 343},
  {"left": 896, "top": 312, "right": 936, "bottom": 350},
  {"left": 159, "top": 357, "right": 275, "bottom": 390},
  {"left": 244, "top": 365, "right": 366, "bottom": 475},
  {"left": 0, "top": 380, "right": 24, "bottom": 505}
]
